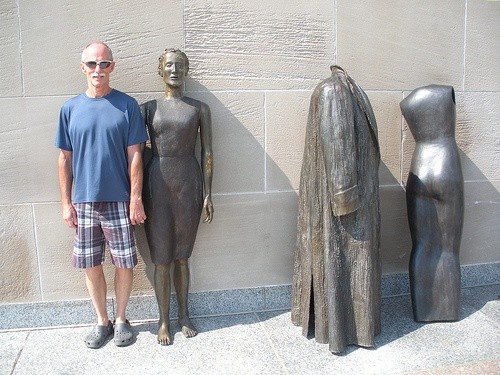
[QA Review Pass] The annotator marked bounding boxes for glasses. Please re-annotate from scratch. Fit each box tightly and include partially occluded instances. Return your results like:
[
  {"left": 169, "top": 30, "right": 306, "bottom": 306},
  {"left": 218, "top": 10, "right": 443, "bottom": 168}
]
[{"left": 82, "top": 60, "right": 112, "bottom": 69}]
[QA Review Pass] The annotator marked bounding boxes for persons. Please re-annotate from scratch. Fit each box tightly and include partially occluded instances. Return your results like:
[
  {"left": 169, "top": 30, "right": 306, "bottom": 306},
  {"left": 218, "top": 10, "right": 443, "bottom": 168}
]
[
  {"left": 53, "top": 41, "right": 149, "bottom": 348},
  {"left": 139, "top": 47, "right": 215, "bottom": 347}
]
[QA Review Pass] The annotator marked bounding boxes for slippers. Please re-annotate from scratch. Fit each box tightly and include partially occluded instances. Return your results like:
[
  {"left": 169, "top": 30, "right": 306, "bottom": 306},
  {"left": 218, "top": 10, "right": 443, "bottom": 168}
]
[
  {"left": 113, "top": 320, "right": 133, "bottom": 346},
  {"left": 84, "top": 319, "right": 112, "bottom": 349}
]
